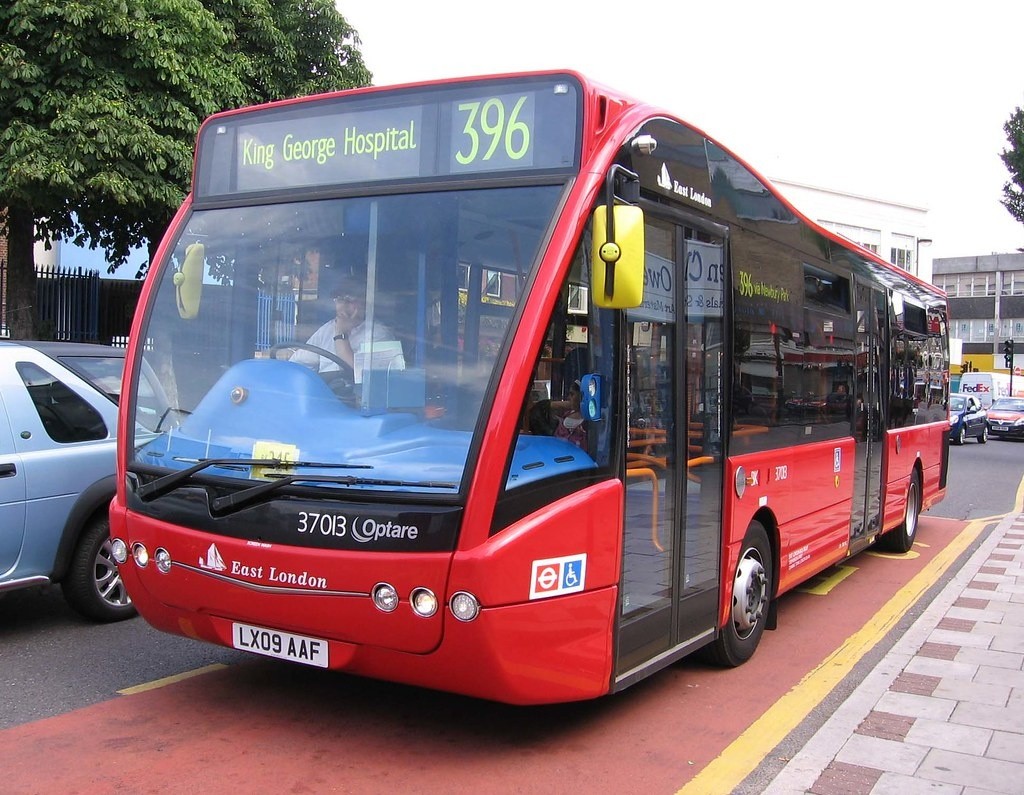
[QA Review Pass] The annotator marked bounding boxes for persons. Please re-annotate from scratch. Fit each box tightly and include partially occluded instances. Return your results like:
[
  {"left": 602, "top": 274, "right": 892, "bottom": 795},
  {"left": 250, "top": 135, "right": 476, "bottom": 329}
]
[
  {"left": 551, "top": 377, "right": 589, "bottom": 453},
  {"left": 289, "top": 276, "right": 397, "bottom": 388}
]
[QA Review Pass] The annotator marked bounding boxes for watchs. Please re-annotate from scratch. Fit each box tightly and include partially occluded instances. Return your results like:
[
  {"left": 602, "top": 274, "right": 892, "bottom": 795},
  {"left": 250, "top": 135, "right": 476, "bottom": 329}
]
[{"left": 333, "top": 333, "right": 348, "bottom": 341}]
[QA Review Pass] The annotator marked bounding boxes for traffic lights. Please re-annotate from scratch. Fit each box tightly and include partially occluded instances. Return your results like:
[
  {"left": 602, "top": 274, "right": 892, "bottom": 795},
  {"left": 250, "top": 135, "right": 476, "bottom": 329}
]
[{"left": 1003, "top": 339, "right": 1014, "bottom": 370}]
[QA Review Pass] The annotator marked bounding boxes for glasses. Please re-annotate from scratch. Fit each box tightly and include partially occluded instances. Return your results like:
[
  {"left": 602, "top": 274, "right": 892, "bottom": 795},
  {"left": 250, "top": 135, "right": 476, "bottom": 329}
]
[{"left": 333, "top": 294, "right": 362, "bottom": 305}]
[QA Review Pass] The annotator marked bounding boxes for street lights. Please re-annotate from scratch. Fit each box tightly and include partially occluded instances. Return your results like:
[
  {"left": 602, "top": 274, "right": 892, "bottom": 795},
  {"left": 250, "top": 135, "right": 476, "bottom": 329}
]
[{"left": 915, "top": 238, "right": 934, "bottom": 277}]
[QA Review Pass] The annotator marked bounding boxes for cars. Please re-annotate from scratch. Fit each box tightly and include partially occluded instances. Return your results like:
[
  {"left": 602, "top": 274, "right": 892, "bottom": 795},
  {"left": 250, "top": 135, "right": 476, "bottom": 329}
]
[
  {"left": 0, "top": 342, "right": 163, "bottom": 628},
  {"left": 987, "top": 396, "right": 1024, "bottom": 439},
  {"left": 947, "top": 393, "right": 989, "bottom": 446},
  {"left": 3, "top": 337, "right": 182, "bottom": 434}
]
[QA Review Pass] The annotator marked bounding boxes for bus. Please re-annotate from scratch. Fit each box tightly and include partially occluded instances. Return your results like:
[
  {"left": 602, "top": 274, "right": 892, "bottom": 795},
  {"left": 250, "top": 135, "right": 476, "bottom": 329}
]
[
  {"left": 959, "top": 372, "right": 1024, "bottom": 409},
  {"left": 107, "top": 66, "right": 952, "bottom": 706}
]
